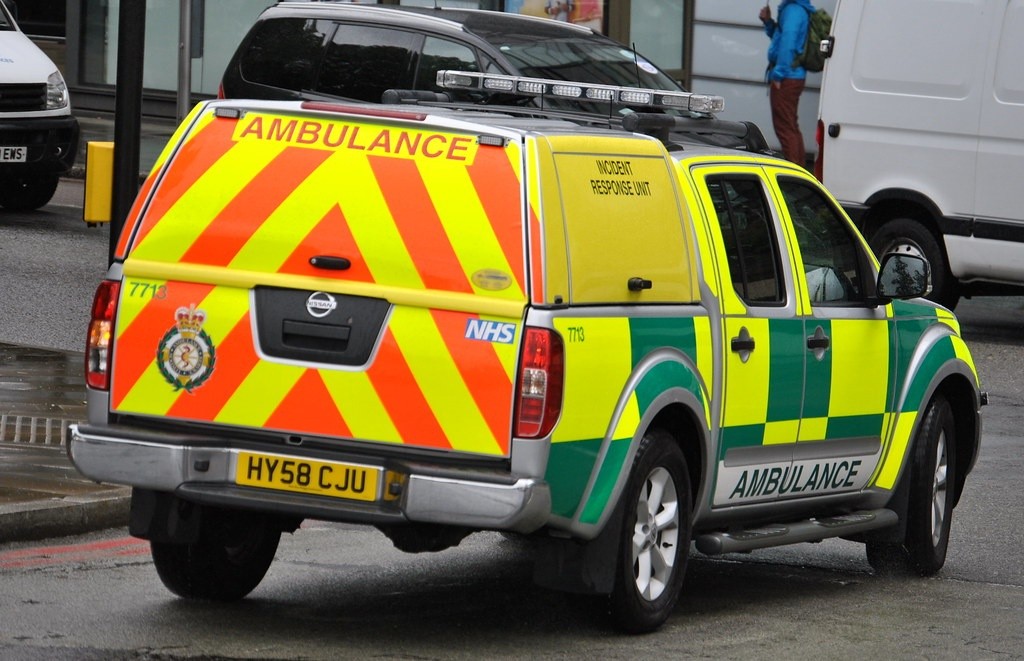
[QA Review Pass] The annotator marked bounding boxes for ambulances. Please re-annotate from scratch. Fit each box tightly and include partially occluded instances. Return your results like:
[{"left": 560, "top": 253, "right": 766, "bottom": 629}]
[{"left": 64, "top": 67, "right": 990, "bottom": 638}]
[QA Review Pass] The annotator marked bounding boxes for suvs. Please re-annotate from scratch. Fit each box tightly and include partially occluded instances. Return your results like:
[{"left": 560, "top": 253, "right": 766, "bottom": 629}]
[{"left": 217, "top": 1, "right": 772, "bottom": 260}]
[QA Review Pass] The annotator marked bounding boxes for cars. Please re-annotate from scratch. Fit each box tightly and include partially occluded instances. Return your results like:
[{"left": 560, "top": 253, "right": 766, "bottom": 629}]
[{"left": 0, "top": 0, "right": 81, "bottom": 215}]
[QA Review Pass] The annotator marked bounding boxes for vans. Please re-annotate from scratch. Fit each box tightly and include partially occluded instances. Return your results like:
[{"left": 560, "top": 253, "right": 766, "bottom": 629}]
[{"left": 812, "top": 0, "right": 1024, "bottom": 311}]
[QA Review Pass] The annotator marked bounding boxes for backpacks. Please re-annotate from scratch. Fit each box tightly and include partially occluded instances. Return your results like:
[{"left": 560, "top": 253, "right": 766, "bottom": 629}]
[{"left": 777, "top": 0, "right": 835, "bottom": 72}]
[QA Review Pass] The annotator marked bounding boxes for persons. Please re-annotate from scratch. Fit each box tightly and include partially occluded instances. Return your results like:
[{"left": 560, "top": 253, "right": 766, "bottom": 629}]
[{"left": 759, "top": 0, "right": 816, "bottom": 167}]
